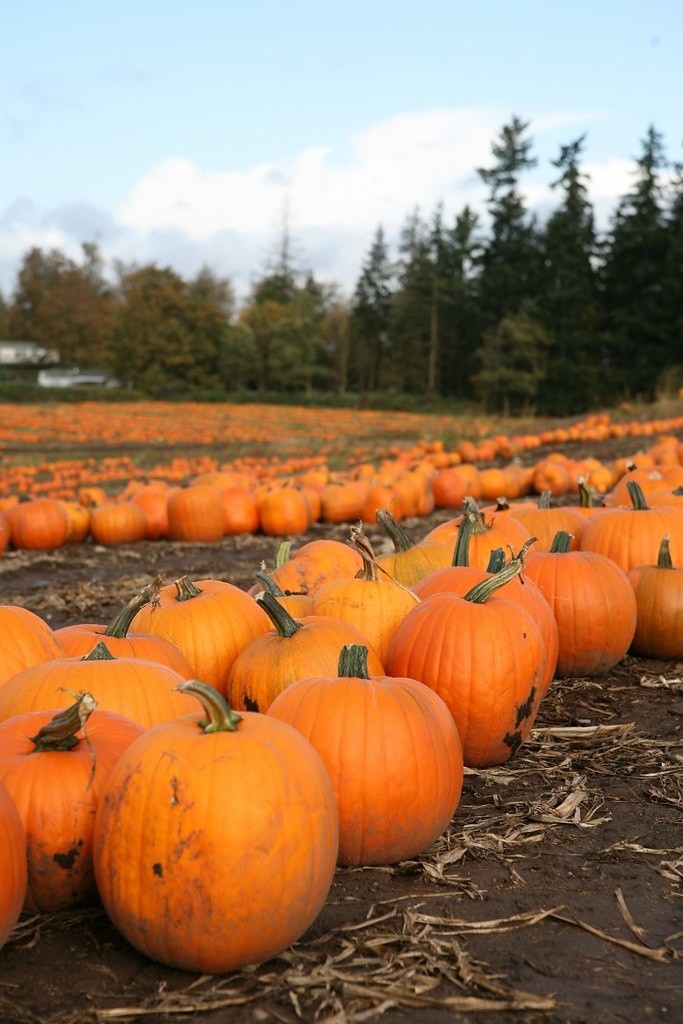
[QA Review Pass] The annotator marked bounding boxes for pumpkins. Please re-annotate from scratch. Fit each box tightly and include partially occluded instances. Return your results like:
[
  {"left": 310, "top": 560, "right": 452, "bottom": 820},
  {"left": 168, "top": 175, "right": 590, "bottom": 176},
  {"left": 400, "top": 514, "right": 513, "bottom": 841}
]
[{"left": 0, "top": 385, "right": 683, "bottom": 965}]
